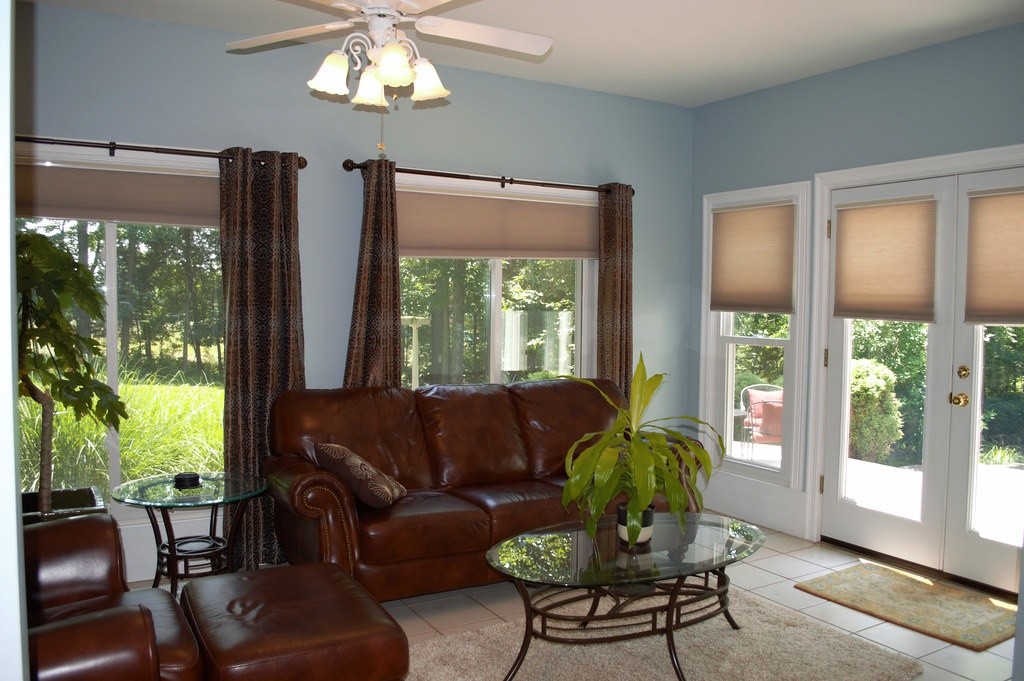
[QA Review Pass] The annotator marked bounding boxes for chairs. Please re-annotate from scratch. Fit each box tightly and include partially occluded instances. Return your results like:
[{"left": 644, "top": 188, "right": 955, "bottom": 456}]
[
  {"left": 746, "top": 401, "right": 784, "bottom": 461},
  {"left": 740, "top": 384, "right": 783, "bottom": 460}
]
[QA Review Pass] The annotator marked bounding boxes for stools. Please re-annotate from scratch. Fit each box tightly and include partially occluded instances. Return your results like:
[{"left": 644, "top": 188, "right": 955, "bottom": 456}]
[{"left": 181, "top": 562, "right": 409, "bottom": 681}]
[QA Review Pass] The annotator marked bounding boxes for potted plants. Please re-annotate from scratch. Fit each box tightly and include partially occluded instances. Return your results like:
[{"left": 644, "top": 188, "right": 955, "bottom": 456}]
[
  {"left": 16, "top": 231, "right": 128, "bottom": 525},
  {"left": 555, "top": 349, "right": 725, "bottom": 551}
]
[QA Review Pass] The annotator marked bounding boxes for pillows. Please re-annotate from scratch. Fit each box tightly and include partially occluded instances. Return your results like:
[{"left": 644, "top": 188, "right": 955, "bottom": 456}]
[
  {"left": 747, "top": 389, "right": 783, "bottom": 418},
  {"left": 313, "top": 442, "right": 407, "bottom": 509},
  {"left": 758, "top": 399, "right": 783, "bottom": 435}
]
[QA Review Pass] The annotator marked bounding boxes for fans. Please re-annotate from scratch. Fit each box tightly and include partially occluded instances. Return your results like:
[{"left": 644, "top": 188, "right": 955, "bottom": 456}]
[{"left": 226, "top": 0, "right": 554, "bottom": 57}]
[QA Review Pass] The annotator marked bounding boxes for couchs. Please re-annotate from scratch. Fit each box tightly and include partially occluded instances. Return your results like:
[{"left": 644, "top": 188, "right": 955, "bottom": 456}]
[
  {"left": 259, "top": 378, "right": 706, "bottom": 604},
  {"left": 24, "top": 513, "right": 203, "bottom": 681}
]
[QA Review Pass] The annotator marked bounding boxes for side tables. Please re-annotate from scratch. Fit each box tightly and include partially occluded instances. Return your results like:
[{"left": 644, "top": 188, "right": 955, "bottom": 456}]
[{"left": 110, "top": 469, "right": 271, "bottom": 599}]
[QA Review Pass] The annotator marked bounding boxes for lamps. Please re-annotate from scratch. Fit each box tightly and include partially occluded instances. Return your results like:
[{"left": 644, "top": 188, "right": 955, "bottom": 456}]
[
  {"left": 350, "top": 64, "right": 389, "bottom": 107},
  {"left": 399, "top": 38, "right": 451, "bottom": 101},
  {"left": 374, "top": 26, "right": 418, "bottom": 87},
  {"left": 307, "top": 32, "right": 372, "bottom": 96}
]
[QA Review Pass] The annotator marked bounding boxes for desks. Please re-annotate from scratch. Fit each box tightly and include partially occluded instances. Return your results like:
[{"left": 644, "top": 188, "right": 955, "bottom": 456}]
[{"left": 735, "top": 408, "right": 749, "bottom": 422}]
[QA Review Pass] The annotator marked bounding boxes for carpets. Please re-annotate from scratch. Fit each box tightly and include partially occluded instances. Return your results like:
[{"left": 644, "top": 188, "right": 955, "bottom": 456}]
[
  {"left": 794, "top": 563, "right": 1017, "bottom": 652},
  {"left": 405, "top": 577, "right": 923, "bottom": 681}
]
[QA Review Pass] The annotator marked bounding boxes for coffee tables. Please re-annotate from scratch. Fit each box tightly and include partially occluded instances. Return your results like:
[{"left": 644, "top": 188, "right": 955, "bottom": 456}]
[{"left": 485, "top": 510, "right": 767, "bottom": 681}]
